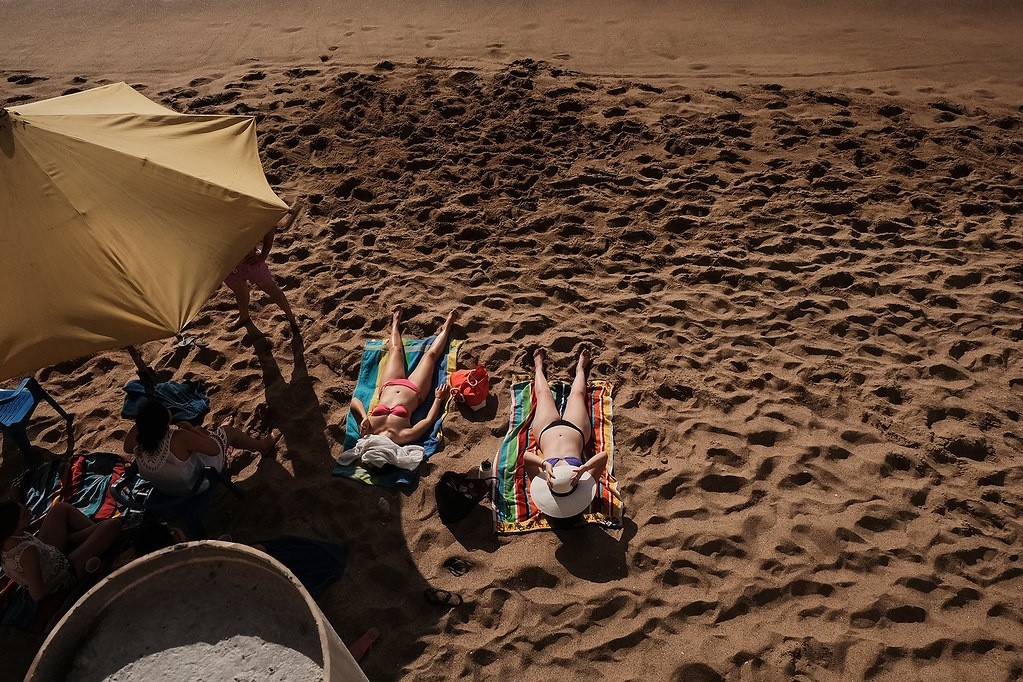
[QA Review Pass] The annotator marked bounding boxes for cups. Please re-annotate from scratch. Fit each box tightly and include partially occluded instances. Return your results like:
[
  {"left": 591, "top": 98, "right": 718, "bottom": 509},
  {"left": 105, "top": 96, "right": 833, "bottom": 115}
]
[
  {"left": 218, "top": 534, "right": 232, "bottom": 542},
  {"left": 86, "top": 557, "right": 104, "bottom": 578}
]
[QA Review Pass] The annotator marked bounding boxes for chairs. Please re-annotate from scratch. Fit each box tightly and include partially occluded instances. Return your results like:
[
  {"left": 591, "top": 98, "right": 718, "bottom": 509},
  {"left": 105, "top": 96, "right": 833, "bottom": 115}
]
[
  {"left": 0, "top": 377, "right": 68, "bottom": 455},
  {"left": 110, "top": 457, "right": 241, "bottom": 540}
]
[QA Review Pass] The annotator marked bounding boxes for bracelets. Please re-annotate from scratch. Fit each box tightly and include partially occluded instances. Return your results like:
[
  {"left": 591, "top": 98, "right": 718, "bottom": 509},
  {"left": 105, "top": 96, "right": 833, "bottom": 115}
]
[
  {"left": 435, "top": 396, "right": 442, "bottom": 401},
  {"left": 541, "top": 460, "right": 546, "bottom": 469}
]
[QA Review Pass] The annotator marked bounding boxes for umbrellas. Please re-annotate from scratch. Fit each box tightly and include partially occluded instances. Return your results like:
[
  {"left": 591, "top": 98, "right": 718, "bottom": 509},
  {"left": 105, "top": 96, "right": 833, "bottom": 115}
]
[{"left": 0, "top": 80, "right": 295, "bottom": 386}]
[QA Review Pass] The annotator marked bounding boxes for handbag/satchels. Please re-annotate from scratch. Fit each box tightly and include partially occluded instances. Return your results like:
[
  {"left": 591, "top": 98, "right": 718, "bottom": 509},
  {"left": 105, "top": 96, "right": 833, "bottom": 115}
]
[
  {"left": 434, "top": 470, "right": 500, "bottom": 525},
  {"left": 450, "top": 363, "right": 489, "bottom": 410}
]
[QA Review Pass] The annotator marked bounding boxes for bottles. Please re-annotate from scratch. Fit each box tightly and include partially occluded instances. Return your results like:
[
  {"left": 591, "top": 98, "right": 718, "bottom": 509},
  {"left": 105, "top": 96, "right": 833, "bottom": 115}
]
[{"left": 478, "top": 458, "right": 492, "bottom": 486}]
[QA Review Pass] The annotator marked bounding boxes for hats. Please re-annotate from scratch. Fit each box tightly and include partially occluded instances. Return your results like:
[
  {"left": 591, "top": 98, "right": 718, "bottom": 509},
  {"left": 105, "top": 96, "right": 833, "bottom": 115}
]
[{"left": 529, "top": 466, "right": 597, "bottom": 518}]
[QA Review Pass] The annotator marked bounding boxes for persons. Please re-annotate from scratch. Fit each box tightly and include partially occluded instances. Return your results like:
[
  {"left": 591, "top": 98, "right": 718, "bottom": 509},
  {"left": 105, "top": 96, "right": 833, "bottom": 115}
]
[
  {"left": 350, "top": 304, "right": 458, "bottom": 471},
  {"left": 223, "top": 225, "right": 302, "bottom": 331},
  {"left": 0, "top": 499, "right": 123, "bottom": 601},
  {"left": 124, "top": 398, "right": 282, "bottom": 497},
  {"left": 522, "top": 347, "right": 609, "bottom": 519}
]
